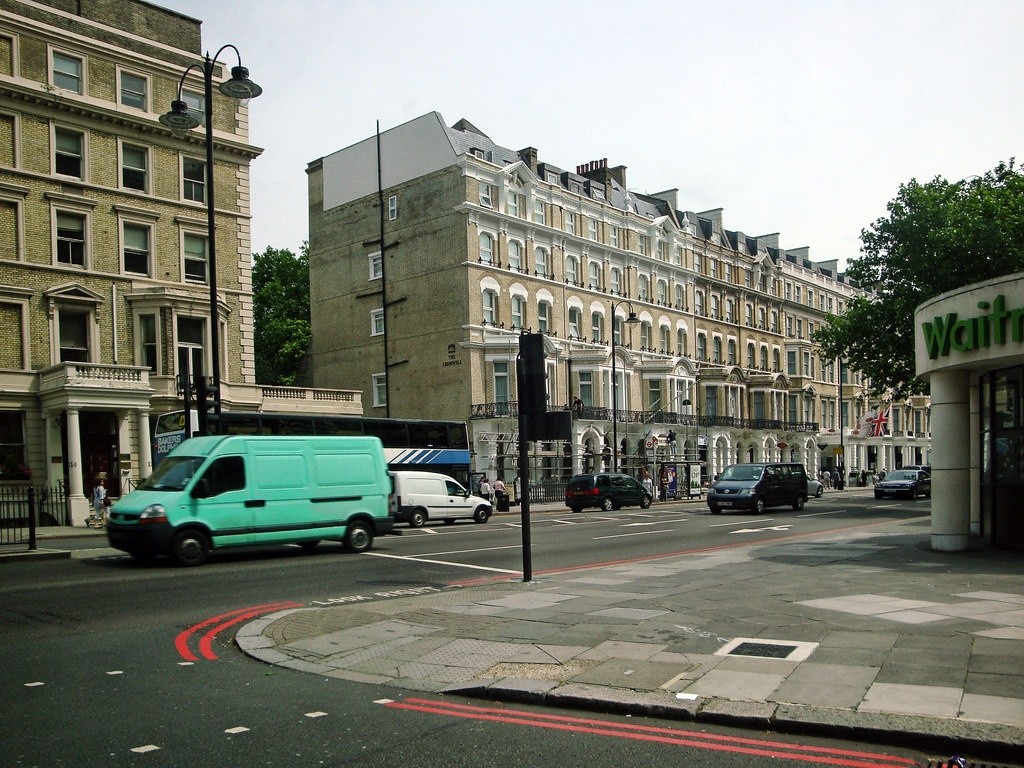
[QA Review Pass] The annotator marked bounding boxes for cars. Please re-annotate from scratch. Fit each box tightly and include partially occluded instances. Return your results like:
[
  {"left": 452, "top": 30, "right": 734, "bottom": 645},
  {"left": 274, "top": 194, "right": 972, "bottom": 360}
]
[
  {"left": 792, "top": 471, "right": 823, "bottom": 497},
  {"left": 874, "top": 470, "right": 931, "bottom": 500},
  {"left": 904, "top": 465, "right": 931, "bottom": 470}
]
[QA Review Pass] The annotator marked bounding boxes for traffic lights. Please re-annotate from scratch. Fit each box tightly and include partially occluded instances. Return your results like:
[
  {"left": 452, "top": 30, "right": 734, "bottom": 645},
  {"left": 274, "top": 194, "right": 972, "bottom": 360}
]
[
  {"left": 205, "top": 386, "right": 219, "bottom": 423},
  {"left": 519, "top": 334, "right": 550, "bottom": 413}
]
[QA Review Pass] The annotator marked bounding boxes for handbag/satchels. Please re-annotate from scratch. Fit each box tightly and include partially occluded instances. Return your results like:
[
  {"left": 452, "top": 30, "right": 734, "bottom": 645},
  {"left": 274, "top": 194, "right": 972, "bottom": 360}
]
[
  {"left": 663, "top": 485, "right": 669, "bottom": 491},
  {"left": 103, "top": 497, "right": 111, "bottom": 507}
]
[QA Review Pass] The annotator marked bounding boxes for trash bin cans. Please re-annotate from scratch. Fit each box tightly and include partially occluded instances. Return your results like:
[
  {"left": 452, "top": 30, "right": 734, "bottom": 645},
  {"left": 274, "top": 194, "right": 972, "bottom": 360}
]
[
  {"left": 498, "top": 494, "right": 509, "bottom": 512},
  {"left": 849, "top": 472, "right": 859, "bottom": 486}
]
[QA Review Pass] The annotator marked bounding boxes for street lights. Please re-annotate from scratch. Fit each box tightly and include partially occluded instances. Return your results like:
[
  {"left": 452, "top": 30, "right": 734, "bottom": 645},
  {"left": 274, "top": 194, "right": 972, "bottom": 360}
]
[
  {"left": 158, "top": 44, "right": 262, "bottom": 435},
  {"left": 611, "top": 300, "right": 642, "bottom": 473}
]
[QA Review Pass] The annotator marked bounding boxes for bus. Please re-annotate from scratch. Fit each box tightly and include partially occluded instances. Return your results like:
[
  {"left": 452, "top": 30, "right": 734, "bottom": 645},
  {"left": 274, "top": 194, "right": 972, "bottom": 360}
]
[{"left": 154, "top": 409, "right": 473, "bottom": 495}]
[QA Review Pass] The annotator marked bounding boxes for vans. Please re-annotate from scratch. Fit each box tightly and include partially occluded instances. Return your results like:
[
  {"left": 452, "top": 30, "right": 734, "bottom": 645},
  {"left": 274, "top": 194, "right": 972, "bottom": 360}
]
[
  {"left": 388, "top": 471, "right": 492, "bottom": 527},
  {"left": 707, "top": 462, "right": 808, "bottom": 514},
  {"left": 107, "top": 434, "right": 394, "bottom": 566}
]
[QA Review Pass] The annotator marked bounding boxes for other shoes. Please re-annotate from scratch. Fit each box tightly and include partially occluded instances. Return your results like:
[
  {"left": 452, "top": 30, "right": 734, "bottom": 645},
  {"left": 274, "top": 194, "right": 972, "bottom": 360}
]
[
  {"left": 85, "top": 519, "right": 91, "bottom": 527},
  {"left": 94, "top": 526, "right": 102, "bottom": 529}
]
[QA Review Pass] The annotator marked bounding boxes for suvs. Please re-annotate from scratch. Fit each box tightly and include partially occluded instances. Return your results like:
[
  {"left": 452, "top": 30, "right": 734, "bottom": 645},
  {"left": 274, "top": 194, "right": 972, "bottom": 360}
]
[{"left": 565, "top": 473, "right": 653, "bottom": 511}]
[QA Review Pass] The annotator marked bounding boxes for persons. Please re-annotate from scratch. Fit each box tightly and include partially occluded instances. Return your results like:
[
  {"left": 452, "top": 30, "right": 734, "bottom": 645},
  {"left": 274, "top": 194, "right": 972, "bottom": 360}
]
[
  {"left": 478, "top": 476, "right": 491, "bottom": 502},
  {"left": 84, "top": 474, "right": 113, "bottom": 529},
  {"left": 642, "top": 474, "right": 653, "bottom": 494},
  {"left": 573, "top": 396, "right": 585, "bottom": 419},
  {"left": 861, "top": 469, "right": 867, "bottom": 487},
  {"left": 880, "top": 468, "right": 887, "bottom": 479},
  {"left": 822, "top": 469, "right": 831, "bottom": 489},
  {"left": 492, "top": 475, "right": 510, "bottom": 511},
  {"left": 666, "top": 429, "right": 677, "bottom": 455},
  {"left": 659, "top": 472, "right": 669, "bottom": 503},
  {"left": 831, "top": 468, "right": 840, "bottom": 490}
]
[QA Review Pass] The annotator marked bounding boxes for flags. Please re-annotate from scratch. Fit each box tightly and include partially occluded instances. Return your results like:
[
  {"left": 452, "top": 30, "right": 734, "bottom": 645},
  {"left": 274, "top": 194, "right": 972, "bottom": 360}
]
[
  {"left": 866, "top": 396, "right": 891, "bottom": 438},
  {"left": 857, "top": 401, "right": 880, "bottom": 440}
]
